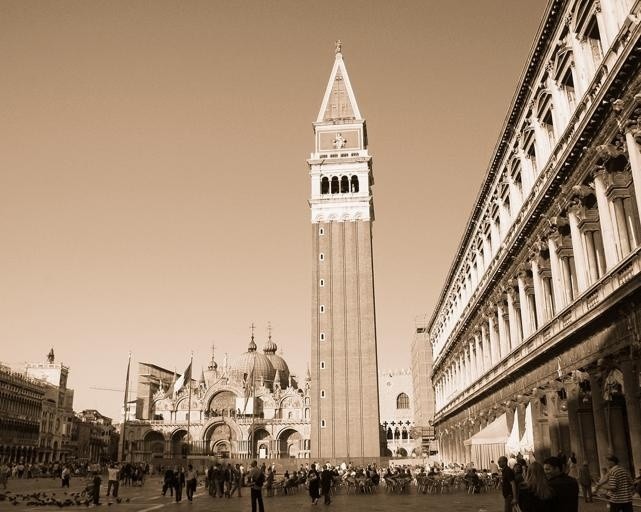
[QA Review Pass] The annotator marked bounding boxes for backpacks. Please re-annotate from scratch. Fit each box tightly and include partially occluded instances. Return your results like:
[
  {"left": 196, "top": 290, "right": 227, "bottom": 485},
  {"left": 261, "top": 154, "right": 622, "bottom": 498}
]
[{"left": 254, "top": 469, "right": 263, "bottom": 487}]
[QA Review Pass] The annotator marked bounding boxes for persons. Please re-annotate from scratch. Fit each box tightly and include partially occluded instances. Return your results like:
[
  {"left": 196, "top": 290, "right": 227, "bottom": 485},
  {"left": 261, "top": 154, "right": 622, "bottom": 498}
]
[{"left": 1, "top": 454, "right": 641, "bottom": 512}]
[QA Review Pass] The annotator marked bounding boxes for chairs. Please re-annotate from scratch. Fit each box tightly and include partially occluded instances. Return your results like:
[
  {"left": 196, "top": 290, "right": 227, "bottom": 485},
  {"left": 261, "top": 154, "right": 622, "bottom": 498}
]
[{"left": 270, "top": 474, "right": 503, "bottom": 497}]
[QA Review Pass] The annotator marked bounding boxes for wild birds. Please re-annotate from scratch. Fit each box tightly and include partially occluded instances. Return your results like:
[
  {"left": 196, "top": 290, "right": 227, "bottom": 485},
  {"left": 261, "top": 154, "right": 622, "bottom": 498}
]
[{"left": 0, "top": 490, "right": 131, "bottom": 508}]
